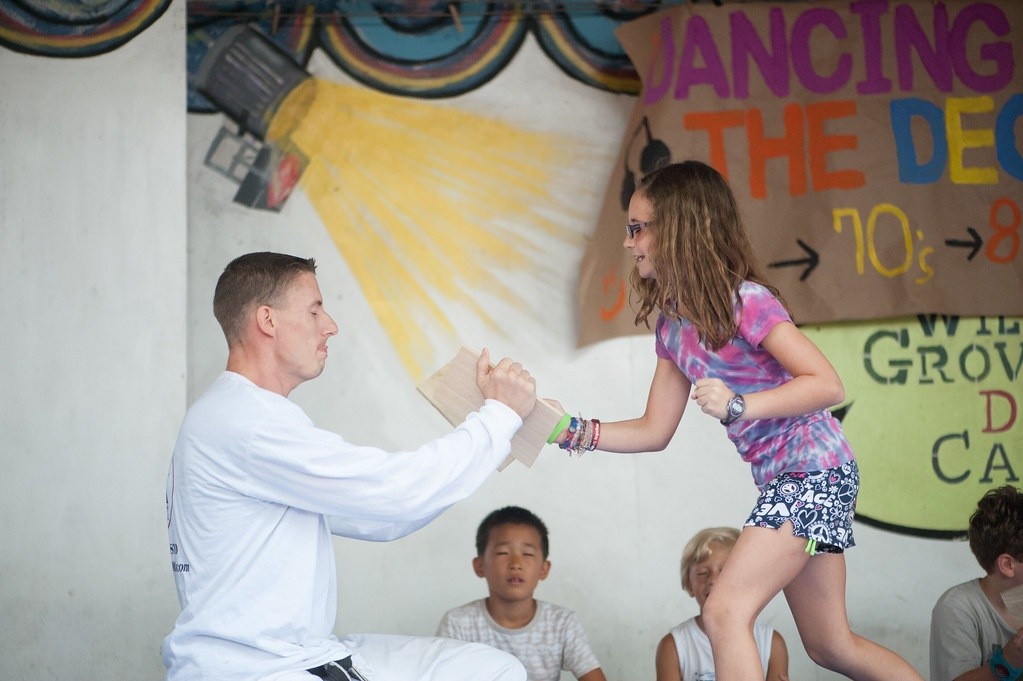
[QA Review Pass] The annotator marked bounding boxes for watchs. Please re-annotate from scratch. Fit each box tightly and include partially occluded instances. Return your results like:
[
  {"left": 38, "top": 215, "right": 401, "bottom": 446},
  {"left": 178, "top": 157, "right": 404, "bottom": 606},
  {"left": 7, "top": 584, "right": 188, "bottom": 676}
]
[
  {"left": 720, "top": 393, "right": 746, "bottom": 426},
  {"left": 989, "top": 648, "right": 1023, "bottom": 681}
]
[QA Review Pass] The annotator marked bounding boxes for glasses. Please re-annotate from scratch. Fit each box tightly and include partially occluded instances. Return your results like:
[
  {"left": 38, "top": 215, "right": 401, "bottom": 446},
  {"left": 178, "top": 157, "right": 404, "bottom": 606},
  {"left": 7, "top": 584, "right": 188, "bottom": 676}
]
[{"left": 625, "top": 220, "right": 655, "bottom": 240}]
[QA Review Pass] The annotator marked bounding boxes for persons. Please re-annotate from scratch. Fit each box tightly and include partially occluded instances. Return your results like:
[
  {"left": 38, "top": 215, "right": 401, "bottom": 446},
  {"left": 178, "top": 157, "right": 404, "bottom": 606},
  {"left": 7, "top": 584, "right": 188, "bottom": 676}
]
[
  {"left": 930, "top": 485, "right": 1023, "bottom": 681},
  {"left": 656, "top": 527, "right": 789, "bottom": 681},
  {"left": 161, "top": 251, "right": 539, "bottom": 681},
  {"left": 434, "top": 507, "right": 607, "bottom": 681},
  {"left": 541, "top": 161, "right": 926, "bottom": 681}
]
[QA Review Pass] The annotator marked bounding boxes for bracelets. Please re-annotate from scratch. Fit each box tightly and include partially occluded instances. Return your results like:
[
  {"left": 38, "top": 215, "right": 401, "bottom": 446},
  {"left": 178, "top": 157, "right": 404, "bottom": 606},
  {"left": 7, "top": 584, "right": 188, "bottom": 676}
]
[{"left": 547, "top": 413, "right": 601, "bottom": 456}]
